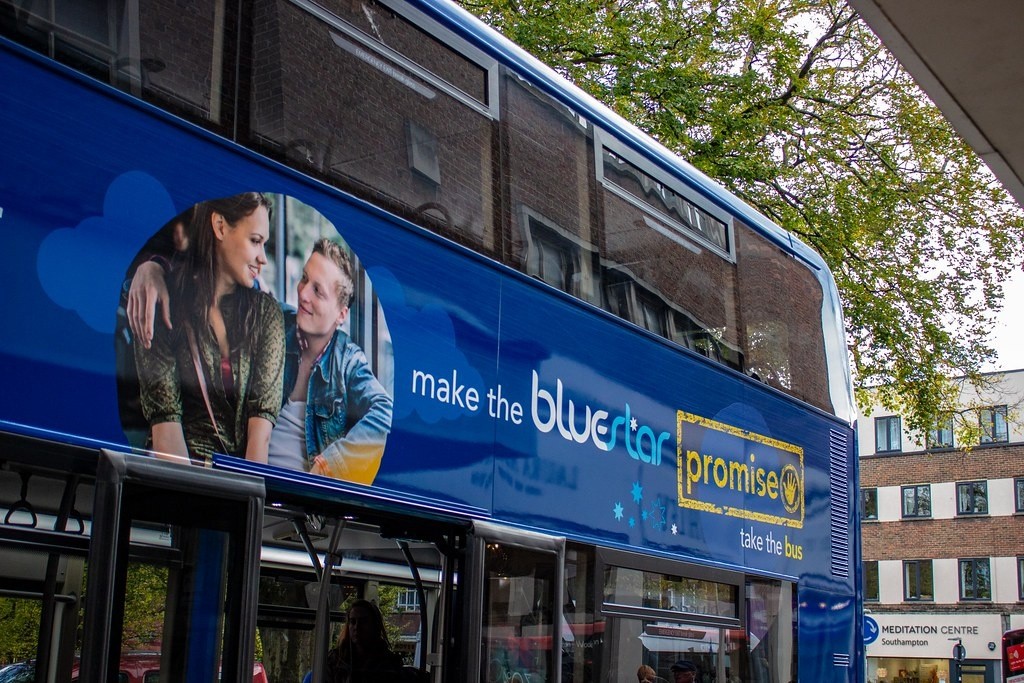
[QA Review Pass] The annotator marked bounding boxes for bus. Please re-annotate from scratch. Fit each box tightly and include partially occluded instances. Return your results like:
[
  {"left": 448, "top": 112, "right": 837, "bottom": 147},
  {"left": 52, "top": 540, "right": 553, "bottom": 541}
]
[{"left": 0, "top": 0, "right": 866, "bottom": 683}]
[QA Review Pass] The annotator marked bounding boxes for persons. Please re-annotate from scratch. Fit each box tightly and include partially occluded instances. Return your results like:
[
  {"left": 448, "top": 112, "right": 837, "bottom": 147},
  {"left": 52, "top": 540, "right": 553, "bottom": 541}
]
[
  {"left": 670, "top": 660, "right": 698, "bottom": 683},
  {"left": 321, "top": 599, "right": 407, "bottom": 683},
  {"left": 637, "top": 664, "right": 656, "bottom": 683},
  {"left": 123, "top": 192, "right": 394, "bottom": 492}
]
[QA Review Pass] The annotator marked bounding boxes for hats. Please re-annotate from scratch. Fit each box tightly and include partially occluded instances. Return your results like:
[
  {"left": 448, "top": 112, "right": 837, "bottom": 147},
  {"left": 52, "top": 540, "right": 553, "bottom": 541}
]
[{"left": 670, "top": 660, "right": 695, "bottom": 669}]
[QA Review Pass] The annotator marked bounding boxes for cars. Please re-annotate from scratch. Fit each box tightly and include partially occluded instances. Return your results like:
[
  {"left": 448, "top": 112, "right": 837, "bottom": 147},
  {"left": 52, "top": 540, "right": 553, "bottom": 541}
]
[{"left": 0, "top": 644, "right": 269, "bottom": 683}]
